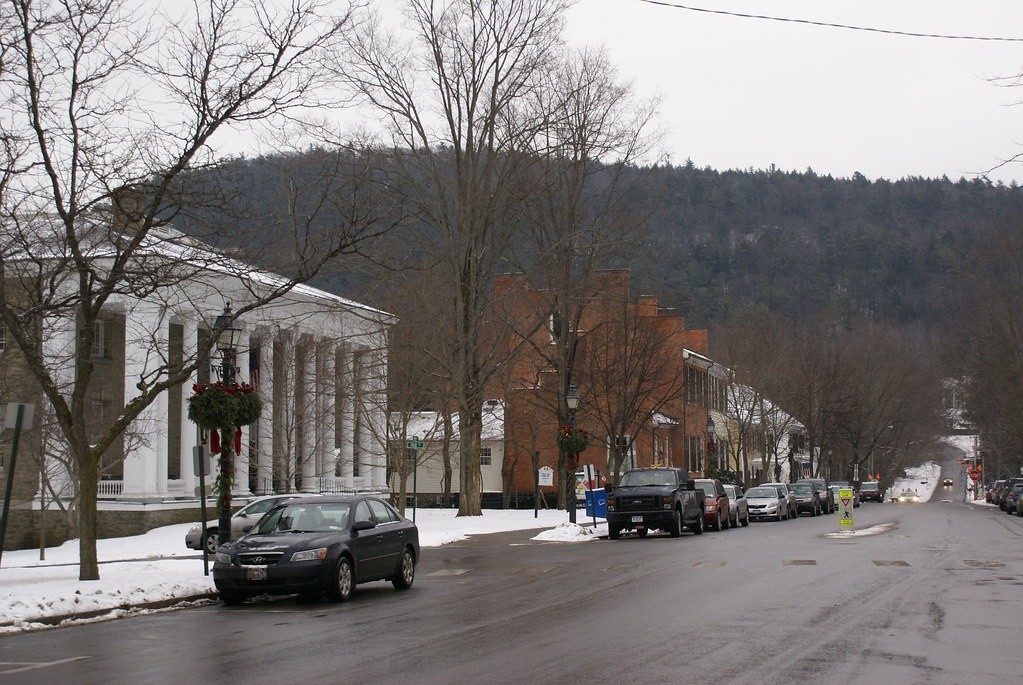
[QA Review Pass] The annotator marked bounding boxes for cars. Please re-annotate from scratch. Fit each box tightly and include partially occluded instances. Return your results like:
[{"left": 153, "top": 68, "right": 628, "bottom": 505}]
[
  {"left": 744, "top": 486, "right": 789, "bottom": 522},
  {"left": 990, "top": 480, "right": 1006, "bottom": 505},
  {"left": 213, "top": 496, "right": 420, "bottom": 607},
  {"left": 943, "top": 476, "right": 953, "bottom": 486},
  {"left": 1016, "top": 487, "right": 1023, "bottom": 517},
  {"left": 985, "top": 482, "right": 995, "bottom": 503},
  {"left": 759, "top": 483, "right": 798, "bottom": 518},
  {"left": 830, "top": 482, "right": 860, "bottom": 510},
  {"left": 789, "top": 483, "right": 822, "bottom": 517},
  {"left": 998, "top": 478, "right": 1023, "bottom": 512},
  {"left": 694, "top": 478, "right": 731, "bottom": 531},
  {"left": 859, "top": 482, "right": 882, "bottom": 503},
  {"left": 185, "top": 494, "right": 349, "bottom": 554},
  {"left": 1006, "top": 483, "right": 1023, "bottom": 515},
  {"left": 796, "top": 479, "right": 835, "bottom": 514},
  {"left": 723, "top": 484, "right": 750, "bottom": 528},
  {"left": 900, "top": 493, "right": 917, "bottom": 503}
]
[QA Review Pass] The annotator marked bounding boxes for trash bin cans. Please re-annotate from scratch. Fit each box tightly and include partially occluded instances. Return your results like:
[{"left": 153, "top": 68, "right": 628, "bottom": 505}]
[{"left": 585, "top": 488, "right": 606, "bottom": 519}]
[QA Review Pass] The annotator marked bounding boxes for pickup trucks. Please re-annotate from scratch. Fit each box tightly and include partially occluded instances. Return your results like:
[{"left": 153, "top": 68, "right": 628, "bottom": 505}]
[{"left": 603, "top": 467, "right": 706, "bottom": 540}]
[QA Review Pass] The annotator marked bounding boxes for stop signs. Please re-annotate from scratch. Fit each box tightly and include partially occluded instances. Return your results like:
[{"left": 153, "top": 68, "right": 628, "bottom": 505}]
[{"left": 970, "top": 470, "right": 981, "bottom": 481}]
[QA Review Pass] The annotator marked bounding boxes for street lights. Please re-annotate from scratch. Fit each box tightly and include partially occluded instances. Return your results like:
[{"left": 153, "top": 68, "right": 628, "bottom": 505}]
[
  {"left": 212, "top": 300, "right": 243, "bottom": 548},
  {"left": 565, "top": 383, "right": 580, "bottom": 523},
  {"left": 871, "top": 426, "right": 893, "bottom": 481},
  {"left": 706, "top": 415, "right": 715, "bottom": 479}
]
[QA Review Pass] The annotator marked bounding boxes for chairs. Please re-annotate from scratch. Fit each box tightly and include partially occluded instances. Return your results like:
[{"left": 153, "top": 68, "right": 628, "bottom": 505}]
[{"left": 297, "top": 511, "right": 323, "bottom": 529}]
